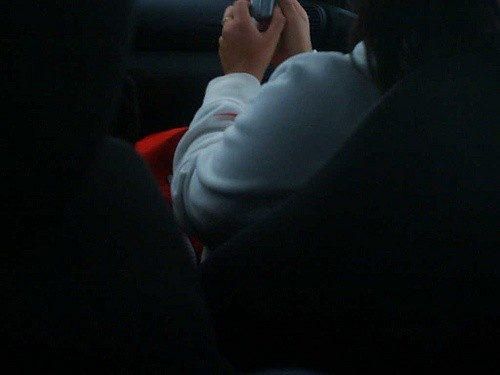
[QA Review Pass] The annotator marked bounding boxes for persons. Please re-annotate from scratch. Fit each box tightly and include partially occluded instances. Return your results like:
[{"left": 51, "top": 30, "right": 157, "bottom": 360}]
[{"left": 133, "top": 0, "right": 500, "bottom": 269}]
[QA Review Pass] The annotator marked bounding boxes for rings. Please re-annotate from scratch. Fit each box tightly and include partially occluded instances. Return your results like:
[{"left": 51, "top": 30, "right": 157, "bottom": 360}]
[{"left": 221, "top": 16, "right": 232, "bottom": 25}]
